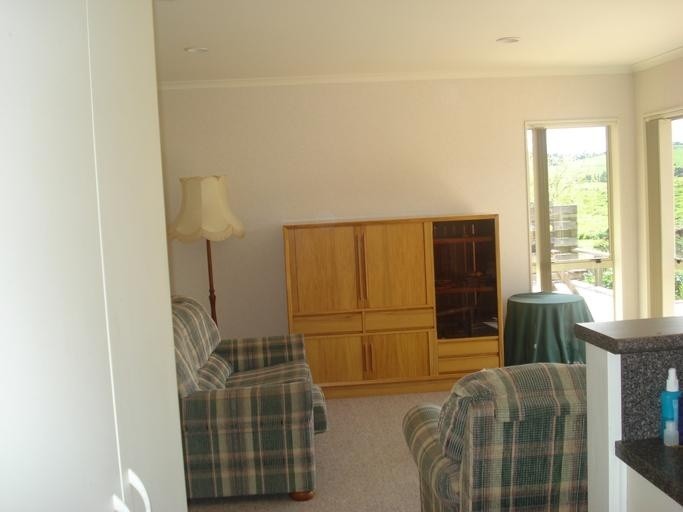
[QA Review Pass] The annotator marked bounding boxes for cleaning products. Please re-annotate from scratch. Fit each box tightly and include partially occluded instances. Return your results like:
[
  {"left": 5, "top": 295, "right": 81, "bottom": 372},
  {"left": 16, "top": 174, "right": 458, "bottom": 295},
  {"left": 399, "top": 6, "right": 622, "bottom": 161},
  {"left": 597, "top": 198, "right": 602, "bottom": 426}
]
[{"left": 659, "top": 367, "right": 681, "bottom": 446}]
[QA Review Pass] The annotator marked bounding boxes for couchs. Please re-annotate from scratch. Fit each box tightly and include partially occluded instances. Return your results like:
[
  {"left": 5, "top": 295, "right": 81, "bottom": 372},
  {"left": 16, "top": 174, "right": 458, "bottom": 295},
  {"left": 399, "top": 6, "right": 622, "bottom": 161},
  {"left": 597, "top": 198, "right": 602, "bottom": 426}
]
[
  {"left": 172, "top": 296, "right": 327, "bottom": 500},
  {"left": 401, "top": 361, "right": 589, "bottom": 511}
]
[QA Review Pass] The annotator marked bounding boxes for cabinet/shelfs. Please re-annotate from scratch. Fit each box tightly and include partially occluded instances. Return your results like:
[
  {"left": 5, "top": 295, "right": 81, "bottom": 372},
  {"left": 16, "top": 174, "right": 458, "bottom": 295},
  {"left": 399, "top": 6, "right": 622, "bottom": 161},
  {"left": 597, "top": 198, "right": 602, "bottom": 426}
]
[{"left": 284, "top": 214, "right": 503, "bottom": 398}]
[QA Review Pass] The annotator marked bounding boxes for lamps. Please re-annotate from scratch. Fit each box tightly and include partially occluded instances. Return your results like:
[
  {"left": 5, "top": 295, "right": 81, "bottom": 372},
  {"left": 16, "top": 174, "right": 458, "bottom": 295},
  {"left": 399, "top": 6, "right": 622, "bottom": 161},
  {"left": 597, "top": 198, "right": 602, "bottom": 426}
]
[{"left": 170, "top": 175, "right": 246, "bottom": 328}]
